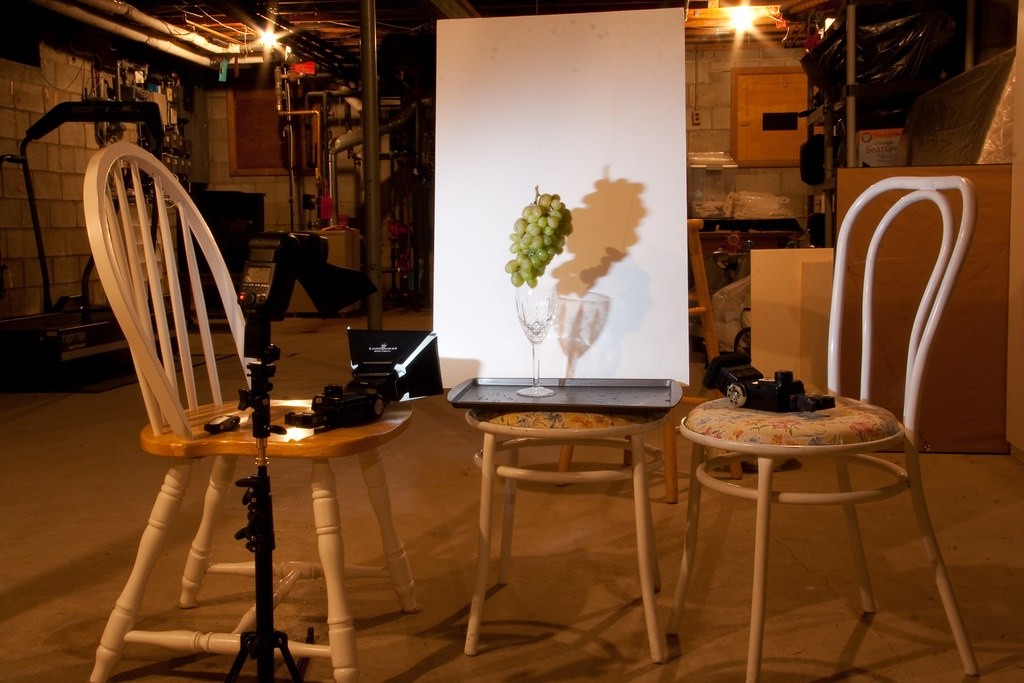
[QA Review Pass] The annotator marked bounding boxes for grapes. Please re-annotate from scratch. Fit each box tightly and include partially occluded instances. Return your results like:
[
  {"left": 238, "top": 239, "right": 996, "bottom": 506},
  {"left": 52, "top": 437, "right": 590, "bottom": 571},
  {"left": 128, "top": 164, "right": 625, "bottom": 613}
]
[{"left": 504, "top": 183, "right": 573, "bottom": 289}]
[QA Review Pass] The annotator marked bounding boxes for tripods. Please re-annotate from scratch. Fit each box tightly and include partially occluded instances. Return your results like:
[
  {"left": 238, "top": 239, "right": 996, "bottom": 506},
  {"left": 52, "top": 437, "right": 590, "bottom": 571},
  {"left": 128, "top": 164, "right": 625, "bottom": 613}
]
[{"left": 223, "top": 308, "right": 305, "bottom": 683}]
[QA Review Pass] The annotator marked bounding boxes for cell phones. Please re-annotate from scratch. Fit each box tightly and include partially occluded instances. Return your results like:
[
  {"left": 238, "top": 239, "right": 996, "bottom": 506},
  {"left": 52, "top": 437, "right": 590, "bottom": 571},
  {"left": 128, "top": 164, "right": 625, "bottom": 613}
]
[{"left": 205, "top": 414, "right": 239, "bottom": 433}]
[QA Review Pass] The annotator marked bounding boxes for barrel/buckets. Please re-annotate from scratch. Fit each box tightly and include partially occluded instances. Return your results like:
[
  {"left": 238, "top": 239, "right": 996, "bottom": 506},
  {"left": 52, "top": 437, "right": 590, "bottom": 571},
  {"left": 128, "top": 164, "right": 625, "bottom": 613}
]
[{"left": 813, "top": 193, "right": 835, "bottom": 213}]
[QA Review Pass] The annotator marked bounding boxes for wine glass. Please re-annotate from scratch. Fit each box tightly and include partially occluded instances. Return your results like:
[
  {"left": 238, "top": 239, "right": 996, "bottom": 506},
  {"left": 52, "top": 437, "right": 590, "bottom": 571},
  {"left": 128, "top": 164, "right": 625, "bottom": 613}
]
[
  {"left": 516, "top": 284, "right": 558, "bottom": 398},
  {"left": 553, "top": 291, "right": 610, "bottom": 379}
]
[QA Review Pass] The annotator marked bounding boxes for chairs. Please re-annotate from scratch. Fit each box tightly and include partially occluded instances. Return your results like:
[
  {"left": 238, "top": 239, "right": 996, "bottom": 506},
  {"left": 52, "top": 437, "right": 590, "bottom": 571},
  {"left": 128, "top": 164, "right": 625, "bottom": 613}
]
[
  {"left": 82, "top": 141, "right": 424, "bottom": 683},
  {"left": 666, "top": 175, "right": 980, "bottom": 683},
  {"left": 462, "top": 405, "right": 664, "bottom": 663}
]
